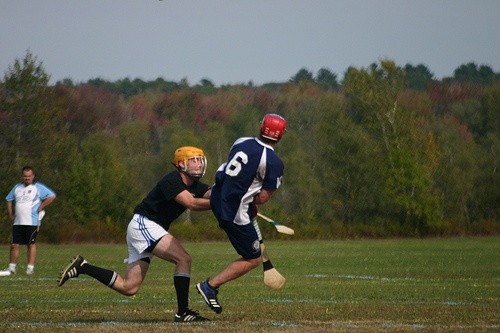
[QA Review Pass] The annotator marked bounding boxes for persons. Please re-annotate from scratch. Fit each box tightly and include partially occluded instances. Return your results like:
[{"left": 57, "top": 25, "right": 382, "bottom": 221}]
[
  {"left": 57, "top": 146, "right": 209, "bottom": 322},
  {"left": 196, "top": 114, "right": 287, "bottom": 314},
  {"left": 3, "top": 166, "right": 56, "bottom": 276}
]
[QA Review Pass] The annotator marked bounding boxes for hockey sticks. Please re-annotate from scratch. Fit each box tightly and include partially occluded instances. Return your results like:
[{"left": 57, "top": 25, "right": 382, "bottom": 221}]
[
  {"left": 251, "top": 215, "right": 286, "bottom": 290},
  {"left": 256, "top": 212, "right": 295, "bottom": 235}
]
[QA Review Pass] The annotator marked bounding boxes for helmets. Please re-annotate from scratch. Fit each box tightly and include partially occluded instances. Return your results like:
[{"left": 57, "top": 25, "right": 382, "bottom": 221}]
[
  {"left": 260, "top": 113, "right": 287, "bottom": 141},
  {"left": 173, "top": 146, "right": 207, "bottom": 178}
]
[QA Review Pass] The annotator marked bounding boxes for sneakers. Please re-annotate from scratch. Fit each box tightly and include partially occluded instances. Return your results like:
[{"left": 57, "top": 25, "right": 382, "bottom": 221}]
[
  {"left": 0, "top": 267, "right": 17, "bottom": 276},
  {"left": 26, "top": 264, "right": 34, "bottom": 275},
  {"left": 196, "top": 278, "right": 222, "bottom": 314},
  {"left": 57, "top": 254, "right": 87, "bottom": 287},
  {"left": 174, "top": 309, "right": 211, "bottom": 322}
]
